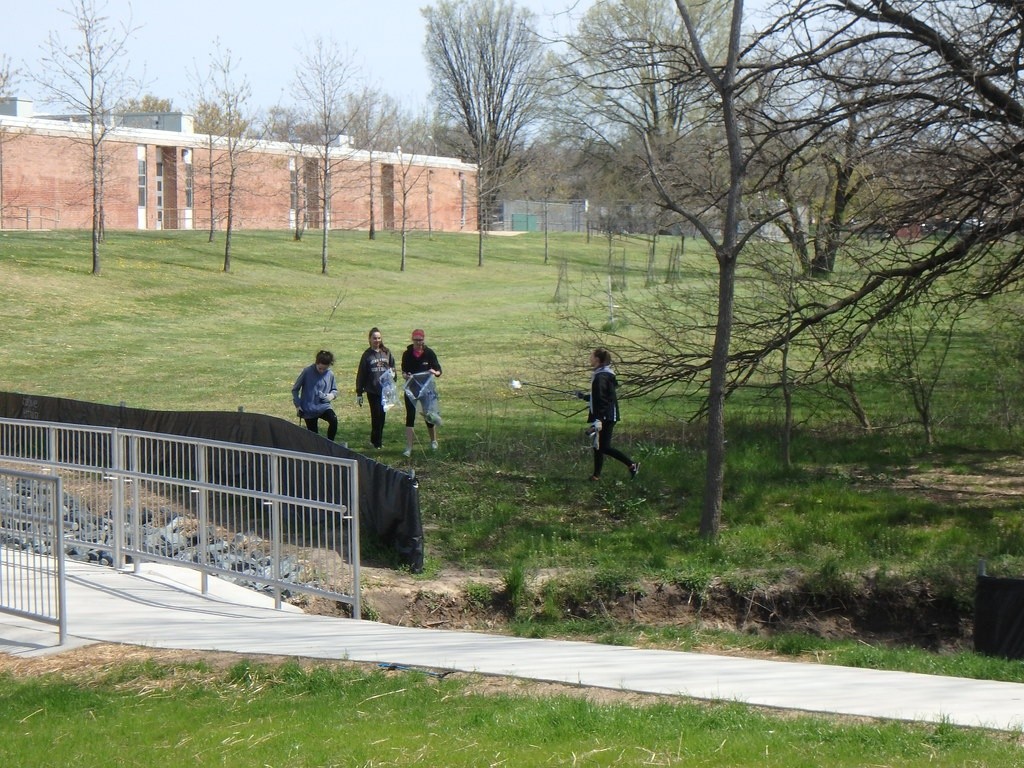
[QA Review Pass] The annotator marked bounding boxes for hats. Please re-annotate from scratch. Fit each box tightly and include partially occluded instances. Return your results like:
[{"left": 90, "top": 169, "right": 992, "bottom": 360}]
[{"left": 412, "top": 329, "right": 425, "bottom": 340}]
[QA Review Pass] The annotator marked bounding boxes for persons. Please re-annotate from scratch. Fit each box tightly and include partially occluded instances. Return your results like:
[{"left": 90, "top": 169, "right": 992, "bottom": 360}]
[
  {"left": 401, "top": 329, "right": 442, "bottom": 458},
  {"left": 355, "top": 327, "right": 397, "bottom": 450},
  {"left": 292, "top": 350, "right": 338, "bottom": 441},
  {"left": 575, "top": 348, "right": 641, "bottom": 482}
]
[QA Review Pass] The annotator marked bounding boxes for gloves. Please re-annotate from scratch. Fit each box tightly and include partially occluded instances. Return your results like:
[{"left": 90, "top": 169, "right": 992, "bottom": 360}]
[
  {"left": 356, "top": 397, "right": 363, "bottom": 407},
  {"left": 571, "top": 389, "right": 585, "bottom": 400},
  {"left": 322, "top": 393, "right": 335, "bottom": 404},
  {"left": 590, "top": 418, "right": 602, "bottom": 431},
  {"left": 295, "top": 406, "right": 304, "bottom": 417}
]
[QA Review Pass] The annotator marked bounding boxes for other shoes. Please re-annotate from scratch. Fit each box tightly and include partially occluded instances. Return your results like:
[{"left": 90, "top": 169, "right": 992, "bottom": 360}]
[
  {"left": 628, "top": 460, "right": 640, "bottom": 482},
  {"left": 582, "top": 475, "right": 601, "bottom": 481},
  {"left": 402, "top": 448, "right": 412, "bottom": 457},
  {"left": 431, "top": 440, "right": 438, "bottom": 451}
]
